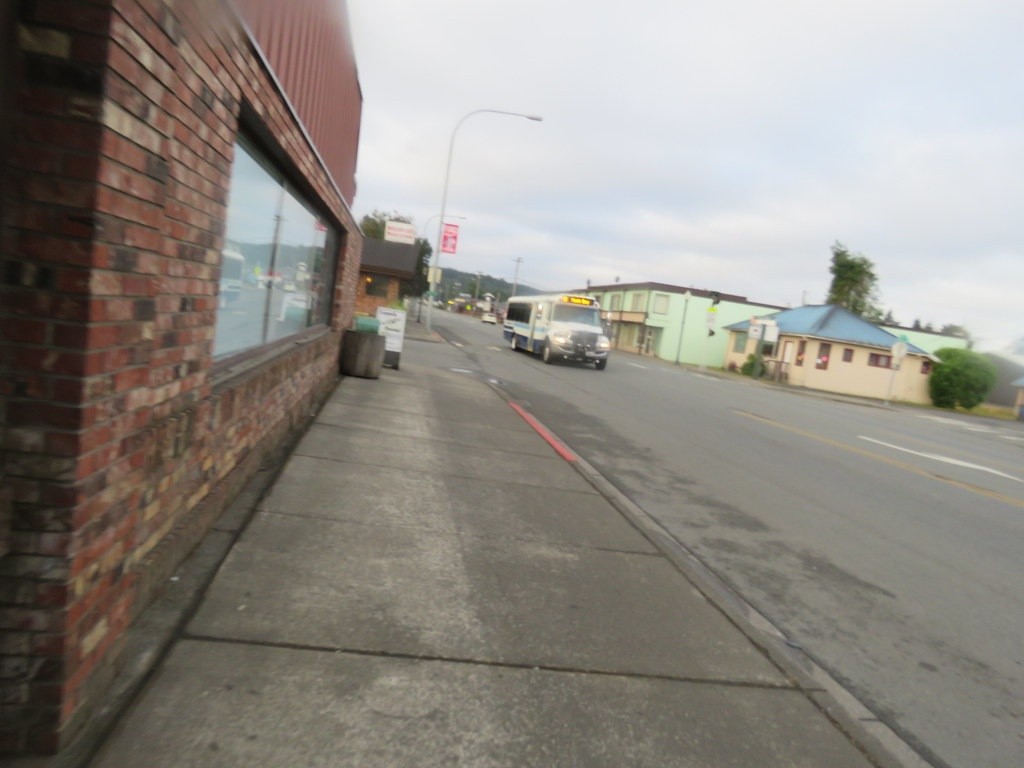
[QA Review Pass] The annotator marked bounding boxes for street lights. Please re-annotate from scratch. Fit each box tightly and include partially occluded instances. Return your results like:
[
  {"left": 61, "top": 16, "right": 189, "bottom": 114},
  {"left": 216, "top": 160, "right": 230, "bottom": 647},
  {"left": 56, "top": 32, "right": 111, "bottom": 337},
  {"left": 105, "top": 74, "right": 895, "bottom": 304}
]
[{"left": 423, "top": 109, "right": 544, "bottom": 335}]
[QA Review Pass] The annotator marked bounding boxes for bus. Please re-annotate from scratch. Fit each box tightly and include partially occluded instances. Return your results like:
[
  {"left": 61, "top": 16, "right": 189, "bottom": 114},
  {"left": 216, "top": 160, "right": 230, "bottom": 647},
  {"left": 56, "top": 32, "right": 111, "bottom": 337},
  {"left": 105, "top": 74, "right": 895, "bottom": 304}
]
[{"left": 503, "top": 295, "right": 611, "bottom": 371}]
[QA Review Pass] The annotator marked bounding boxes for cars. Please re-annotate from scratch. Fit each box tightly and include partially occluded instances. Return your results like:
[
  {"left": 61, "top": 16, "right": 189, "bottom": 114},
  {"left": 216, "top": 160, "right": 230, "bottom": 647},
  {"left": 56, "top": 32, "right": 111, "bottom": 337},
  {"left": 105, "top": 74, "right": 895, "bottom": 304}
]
[{"left": 481, "top": 313, "right": 497, "bottom": 325}]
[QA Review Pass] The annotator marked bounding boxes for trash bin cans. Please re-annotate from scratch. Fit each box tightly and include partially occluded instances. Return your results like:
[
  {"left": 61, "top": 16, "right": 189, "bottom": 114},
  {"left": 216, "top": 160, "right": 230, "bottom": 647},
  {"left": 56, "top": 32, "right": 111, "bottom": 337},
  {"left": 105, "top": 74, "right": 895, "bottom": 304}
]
[{"left": 354, "top": 315, "right": 381, "bottom": 333}]
